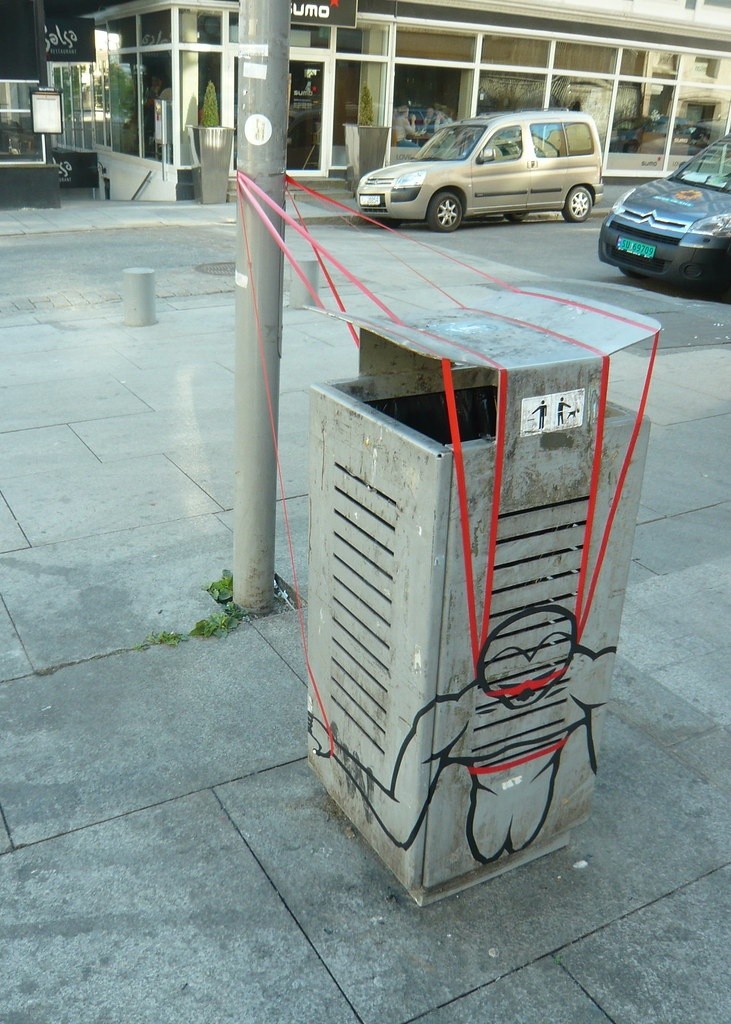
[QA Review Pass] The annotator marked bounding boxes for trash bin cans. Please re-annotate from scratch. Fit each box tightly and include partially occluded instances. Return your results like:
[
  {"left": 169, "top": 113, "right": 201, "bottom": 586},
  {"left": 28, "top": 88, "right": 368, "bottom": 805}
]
[{"left": 289, "top": 286, "right": 667, "bottom": 908}]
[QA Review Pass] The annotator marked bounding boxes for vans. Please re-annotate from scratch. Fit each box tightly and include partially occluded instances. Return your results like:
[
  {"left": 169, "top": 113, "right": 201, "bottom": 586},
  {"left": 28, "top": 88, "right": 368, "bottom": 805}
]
[{"left": 357, "top": 110, "right": 603, "bottom": 232}]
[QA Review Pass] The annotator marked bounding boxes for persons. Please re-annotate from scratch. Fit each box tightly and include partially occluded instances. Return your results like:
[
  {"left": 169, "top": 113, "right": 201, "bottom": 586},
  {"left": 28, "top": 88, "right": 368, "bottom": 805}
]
[
  {"left": 420, "top": 107, "right": 452, "bottom": 134},
  {"left": 144, "top": 74, "right": 169, "bottom": 137},
  {"left": 394, "top": 105, "right": 420, "bottom": 147}
]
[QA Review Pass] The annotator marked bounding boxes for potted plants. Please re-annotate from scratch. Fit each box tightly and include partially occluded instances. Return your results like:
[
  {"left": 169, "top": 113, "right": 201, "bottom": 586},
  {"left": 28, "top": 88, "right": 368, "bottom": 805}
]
[
  {"left": 343, "top": 81, "right": 391, "bottom": 191},
  {"left": 185, "top": 81, "right": 236, "bottom": 204}
]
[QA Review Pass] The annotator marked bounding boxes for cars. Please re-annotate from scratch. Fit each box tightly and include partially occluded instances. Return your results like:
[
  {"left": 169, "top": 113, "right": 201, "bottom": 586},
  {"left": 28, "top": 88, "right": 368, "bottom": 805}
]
[{"left": 598, "top": 134, "right": 729, "bottom": 291}]
